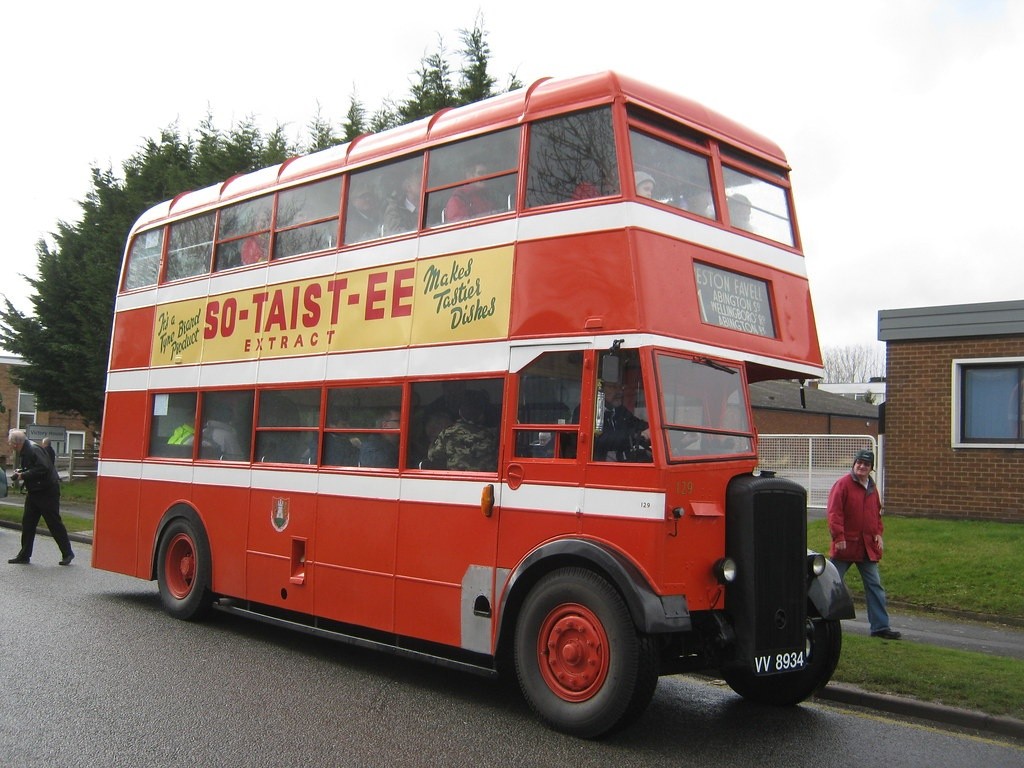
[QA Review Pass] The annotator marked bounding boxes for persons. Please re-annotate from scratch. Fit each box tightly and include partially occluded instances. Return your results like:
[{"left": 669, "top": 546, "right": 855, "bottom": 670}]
[
  {"left": 7, "top": 429, "right": 76, "bottom": 568},
  {"left": 823, "top": 448, "right": 903, "bottom": 641},
  {"left": 205, "top": 203, "right": 322, "bottom": 268},
  {"left": 339, "top": 154, "right": 755, "bottom": 242},
  {"left": 167, "top": 373, "right": 652, "bottom": 473}
]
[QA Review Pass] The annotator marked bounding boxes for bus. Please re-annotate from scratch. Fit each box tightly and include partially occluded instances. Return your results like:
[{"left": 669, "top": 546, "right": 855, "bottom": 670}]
[{"left": 92, "top": 71, "right": 856, "bottom": 739}]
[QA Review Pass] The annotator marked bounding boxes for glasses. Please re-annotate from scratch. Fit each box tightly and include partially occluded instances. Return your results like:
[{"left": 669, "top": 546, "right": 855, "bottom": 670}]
[{"left": 856, "top": 460, "right": 871, "bottom": 467}]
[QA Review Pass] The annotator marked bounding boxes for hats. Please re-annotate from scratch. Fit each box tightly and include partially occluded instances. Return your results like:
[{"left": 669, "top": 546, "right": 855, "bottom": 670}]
[{"left": 853, "top": 449, "right": 876, "bottom": 472}]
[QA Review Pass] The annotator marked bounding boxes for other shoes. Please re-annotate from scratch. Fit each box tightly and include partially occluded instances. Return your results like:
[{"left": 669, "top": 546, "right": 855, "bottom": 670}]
[
  {"left": 8, "top": 557, "right": 31, "bottom": 564},
  {"left": 871, "top": 630, "right": 901, "bottom": 638},
  {"left": 59, "top": 554, "right": 75, "bottom": 565}
]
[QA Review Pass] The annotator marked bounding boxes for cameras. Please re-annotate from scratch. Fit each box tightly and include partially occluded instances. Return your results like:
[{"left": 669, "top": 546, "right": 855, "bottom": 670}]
[{"left": 10, "top": 469, "right": 24, "bottom": 481}]
